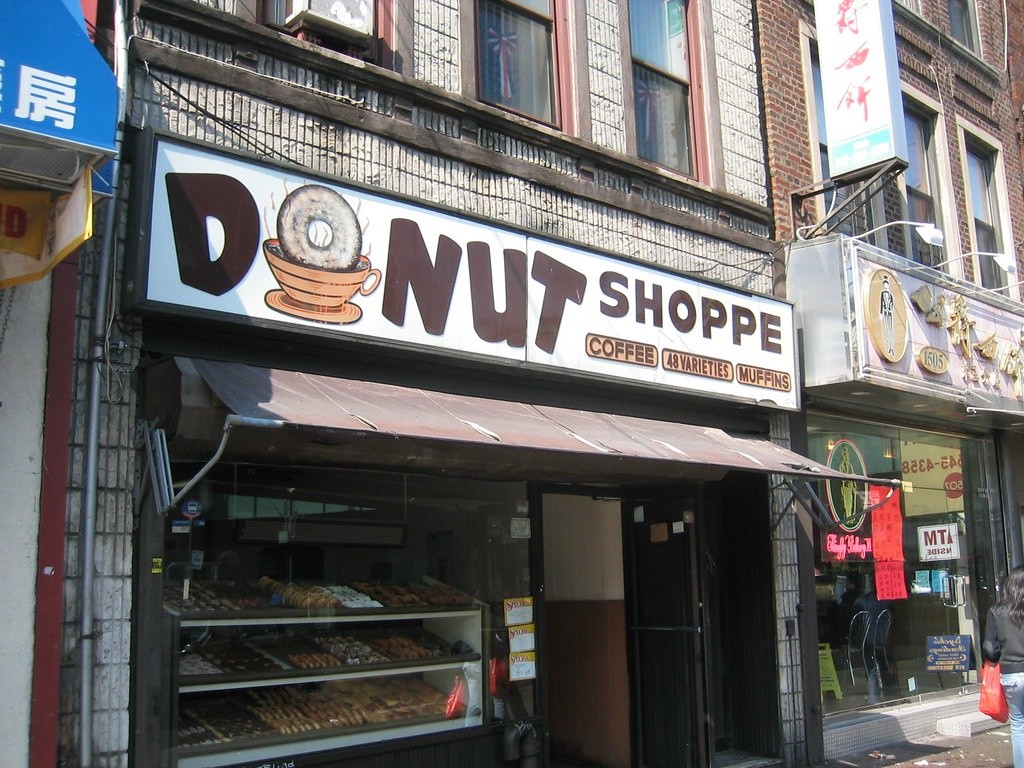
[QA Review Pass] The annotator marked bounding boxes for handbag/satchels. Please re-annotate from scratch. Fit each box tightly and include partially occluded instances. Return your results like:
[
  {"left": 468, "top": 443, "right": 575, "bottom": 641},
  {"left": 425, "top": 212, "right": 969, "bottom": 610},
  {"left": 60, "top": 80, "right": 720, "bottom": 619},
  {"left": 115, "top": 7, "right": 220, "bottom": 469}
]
[{"left": 977, "top": 659, "right": 1008, "bottom": 723}]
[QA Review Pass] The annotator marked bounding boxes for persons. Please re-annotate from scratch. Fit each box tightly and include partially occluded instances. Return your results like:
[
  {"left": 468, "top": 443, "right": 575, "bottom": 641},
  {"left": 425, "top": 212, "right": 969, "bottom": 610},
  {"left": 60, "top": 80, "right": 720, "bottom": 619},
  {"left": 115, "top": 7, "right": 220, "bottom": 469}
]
[
  {"left": 836, "top": 569, "right": 897, "bottom": 644},
  {"left": 982, "top": 565, "right": 1024, "bottom": 768}
]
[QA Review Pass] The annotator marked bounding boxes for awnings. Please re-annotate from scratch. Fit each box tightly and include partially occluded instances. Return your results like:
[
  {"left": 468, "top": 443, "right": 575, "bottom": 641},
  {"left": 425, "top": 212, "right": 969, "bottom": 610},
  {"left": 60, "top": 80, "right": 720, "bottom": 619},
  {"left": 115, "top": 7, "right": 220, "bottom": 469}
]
[{"left": 136, "top": 357, "right": 903, "bottom": 531}]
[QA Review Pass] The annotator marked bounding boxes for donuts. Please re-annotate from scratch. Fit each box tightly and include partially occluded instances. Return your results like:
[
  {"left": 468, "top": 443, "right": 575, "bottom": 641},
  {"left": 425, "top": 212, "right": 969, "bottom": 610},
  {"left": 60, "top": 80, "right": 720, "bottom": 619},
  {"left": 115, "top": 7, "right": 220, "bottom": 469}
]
[{"left": 160, "top": 578, "right": 340, "bottom": 751}]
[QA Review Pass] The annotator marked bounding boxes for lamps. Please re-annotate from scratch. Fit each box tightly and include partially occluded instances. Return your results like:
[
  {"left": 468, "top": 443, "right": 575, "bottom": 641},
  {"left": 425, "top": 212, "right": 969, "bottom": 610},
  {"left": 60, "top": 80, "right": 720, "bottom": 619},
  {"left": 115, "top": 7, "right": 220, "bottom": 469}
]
[
  {"left": 929, "top": 252, "right": 1017, "bottom": 275},
  {"left": 852, "top": 220, "right": 944, "bottom": 248}
]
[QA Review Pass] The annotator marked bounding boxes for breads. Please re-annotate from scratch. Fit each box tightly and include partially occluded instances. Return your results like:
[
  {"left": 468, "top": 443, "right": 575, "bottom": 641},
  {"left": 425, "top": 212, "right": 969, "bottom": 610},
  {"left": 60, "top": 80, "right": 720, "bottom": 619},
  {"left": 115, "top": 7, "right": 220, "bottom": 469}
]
[{"left": 248, "top": 580, "right": 474, "bottom": 735}]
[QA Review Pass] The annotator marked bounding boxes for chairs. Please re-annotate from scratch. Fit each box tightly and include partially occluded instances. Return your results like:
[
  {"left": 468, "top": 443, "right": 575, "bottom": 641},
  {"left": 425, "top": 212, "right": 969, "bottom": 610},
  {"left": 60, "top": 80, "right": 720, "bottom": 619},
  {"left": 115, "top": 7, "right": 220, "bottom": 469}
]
[
  {"left": 865, "top": 609, "right": 891, "bottom": 675},
  {"left": 839, "top": 611, "right": 872, "bottom": 687}
]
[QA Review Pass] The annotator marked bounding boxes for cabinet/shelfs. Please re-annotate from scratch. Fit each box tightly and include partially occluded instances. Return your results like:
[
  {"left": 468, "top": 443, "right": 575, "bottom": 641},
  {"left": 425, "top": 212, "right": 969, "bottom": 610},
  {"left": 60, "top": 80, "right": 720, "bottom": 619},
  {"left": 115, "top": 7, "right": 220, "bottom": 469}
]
[{"left": 163, "top": 574, "right": 494, "bottom": 768}]
